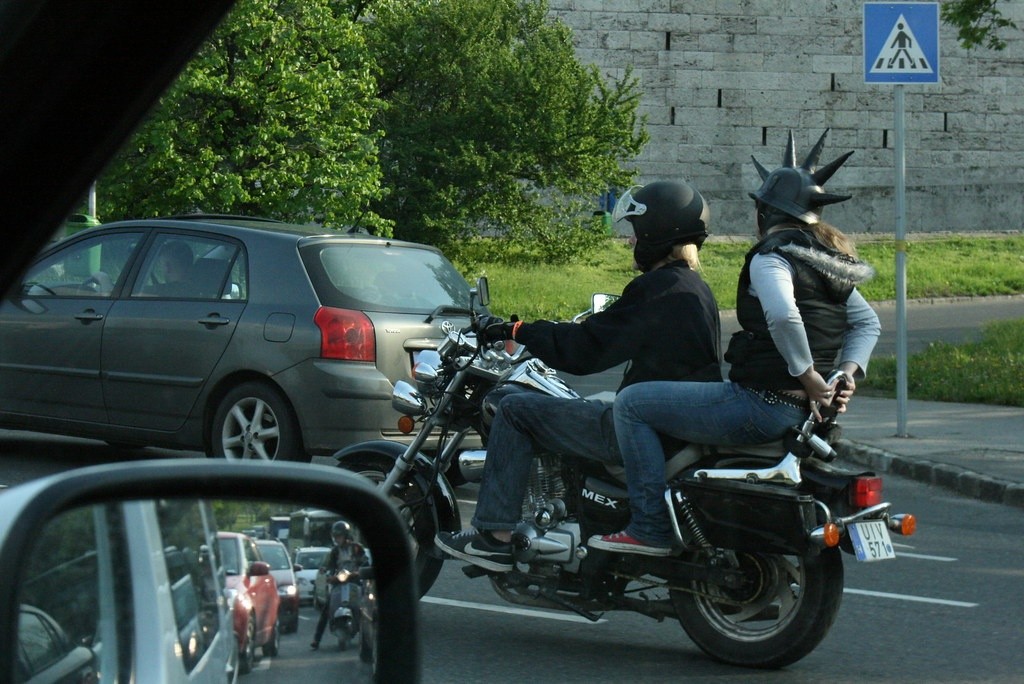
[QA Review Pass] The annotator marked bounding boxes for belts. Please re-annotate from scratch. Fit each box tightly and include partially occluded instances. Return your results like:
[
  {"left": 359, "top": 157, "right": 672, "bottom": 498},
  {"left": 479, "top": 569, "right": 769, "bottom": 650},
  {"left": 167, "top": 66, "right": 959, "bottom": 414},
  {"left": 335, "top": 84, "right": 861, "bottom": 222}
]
[{"left": 747, "top": 386, "right": 810, "bottom": 410}]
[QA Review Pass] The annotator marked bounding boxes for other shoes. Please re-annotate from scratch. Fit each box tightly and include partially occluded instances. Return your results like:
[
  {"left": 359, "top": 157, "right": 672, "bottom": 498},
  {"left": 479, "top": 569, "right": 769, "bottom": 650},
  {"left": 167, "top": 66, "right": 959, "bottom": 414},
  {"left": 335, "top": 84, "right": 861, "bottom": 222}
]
[{"left": 311, "top": 642, "right": 319, "bottom": 649}]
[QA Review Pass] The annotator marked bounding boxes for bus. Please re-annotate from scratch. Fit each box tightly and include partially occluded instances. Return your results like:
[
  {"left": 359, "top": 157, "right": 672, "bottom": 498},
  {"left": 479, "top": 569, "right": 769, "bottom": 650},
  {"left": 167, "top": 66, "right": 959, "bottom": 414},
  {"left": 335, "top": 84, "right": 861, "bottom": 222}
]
[{"left": 287, "top": 508, "right": 360, "bottom": 554}]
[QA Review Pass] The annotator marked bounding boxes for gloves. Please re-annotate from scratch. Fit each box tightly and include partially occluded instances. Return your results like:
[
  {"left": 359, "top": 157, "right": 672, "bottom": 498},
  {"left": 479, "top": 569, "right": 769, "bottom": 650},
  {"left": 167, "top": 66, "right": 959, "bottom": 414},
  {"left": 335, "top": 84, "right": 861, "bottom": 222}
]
[{"left": 477, "top": 315, "right": 519, "bottom": 349}]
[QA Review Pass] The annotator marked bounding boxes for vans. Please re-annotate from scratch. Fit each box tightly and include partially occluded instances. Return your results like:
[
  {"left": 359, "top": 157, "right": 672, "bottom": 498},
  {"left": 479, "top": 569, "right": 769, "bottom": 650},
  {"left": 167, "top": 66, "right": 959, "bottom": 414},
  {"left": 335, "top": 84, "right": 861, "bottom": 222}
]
[
  {"left": 269, "top": 516, "right": 291, "bottom": 545},
  {"left": 19, "top": 498, "right": 240, "bottom": 684}
]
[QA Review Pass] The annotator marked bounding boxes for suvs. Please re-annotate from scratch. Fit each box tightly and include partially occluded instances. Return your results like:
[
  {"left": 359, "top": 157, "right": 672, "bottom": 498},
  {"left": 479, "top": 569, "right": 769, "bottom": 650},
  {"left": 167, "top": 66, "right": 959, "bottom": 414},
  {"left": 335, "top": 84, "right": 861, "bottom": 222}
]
[
  {"left": 252, "top": 537, "right": 303, "bottom": 635},
  {"left": 20, "top": 496, "right": 244, "bottom": 684},
  {"left": 0, "top": 212, "right": 511, "bottom": 463}
]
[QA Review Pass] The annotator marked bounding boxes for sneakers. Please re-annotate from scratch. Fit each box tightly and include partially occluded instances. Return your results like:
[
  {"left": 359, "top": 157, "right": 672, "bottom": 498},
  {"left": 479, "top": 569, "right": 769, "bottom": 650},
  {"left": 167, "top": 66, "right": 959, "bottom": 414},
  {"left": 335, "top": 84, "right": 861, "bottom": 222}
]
[
  {"left": 434, "top": 527, "right": 514, "bottom": 572},
  {"left": 587, "top": 531, "right": 672, "bottom": 557}
]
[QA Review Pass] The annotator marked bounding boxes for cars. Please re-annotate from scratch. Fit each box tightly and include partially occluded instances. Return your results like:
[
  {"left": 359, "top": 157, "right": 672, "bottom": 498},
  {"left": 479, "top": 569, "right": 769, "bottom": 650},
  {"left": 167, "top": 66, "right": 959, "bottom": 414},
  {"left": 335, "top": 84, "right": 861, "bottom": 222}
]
[
  {"left": 292, "top": 547, "right": 334, "bottom": 602},
  {"left": 242, "top": 525, "right": 266, "bottom": 539},
  {"left": 213, "top": 531, "right": 279, "bottom": 674},
  {"left": 356, "top": 550, "right": 376, "bottom": 664}
]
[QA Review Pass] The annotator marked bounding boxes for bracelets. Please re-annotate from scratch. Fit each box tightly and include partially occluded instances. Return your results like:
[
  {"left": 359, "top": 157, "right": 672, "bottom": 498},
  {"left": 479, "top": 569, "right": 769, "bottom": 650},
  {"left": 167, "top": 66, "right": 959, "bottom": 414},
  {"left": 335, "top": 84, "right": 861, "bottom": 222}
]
[{"left": 512, "top": 319, "right": 525, "bottom": 339}]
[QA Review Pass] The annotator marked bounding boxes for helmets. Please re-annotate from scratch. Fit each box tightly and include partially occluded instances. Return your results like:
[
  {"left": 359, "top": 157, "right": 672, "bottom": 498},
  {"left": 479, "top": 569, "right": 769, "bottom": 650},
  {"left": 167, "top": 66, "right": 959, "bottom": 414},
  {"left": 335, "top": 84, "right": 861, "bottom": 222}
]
[
  {"left": 748, "top": 127, "right": 855, "bottom": 224},
  {"left": 332, "top": 521, "right": 350, "bottom": 535},
  {"left": 611, "top": 180, "right": 710, "bottom": 273}
]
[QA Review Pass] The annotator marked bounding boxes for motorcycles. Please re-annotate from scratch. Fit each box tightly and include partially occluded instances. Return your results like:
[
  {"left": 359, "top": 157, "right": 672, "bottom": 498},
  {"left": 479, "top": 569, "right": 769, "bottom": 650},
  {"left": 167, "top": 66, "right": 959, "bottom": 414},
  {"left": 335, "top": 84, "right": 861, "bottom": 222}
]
[{"left": 325, "top": 276, "right": 915, "bottom": 670}]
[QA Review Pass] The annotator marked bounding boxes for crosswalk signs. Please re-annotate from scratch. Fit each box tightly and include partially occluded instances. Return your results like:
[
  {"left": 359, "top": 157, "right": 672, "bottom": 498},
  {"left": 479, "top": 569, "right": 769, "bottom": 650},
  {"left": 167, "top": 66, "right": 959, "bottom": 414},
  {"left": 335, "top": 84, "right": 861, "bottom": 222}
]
[{"left": 862, "top": 1, "right": 941, "bottom": 86}]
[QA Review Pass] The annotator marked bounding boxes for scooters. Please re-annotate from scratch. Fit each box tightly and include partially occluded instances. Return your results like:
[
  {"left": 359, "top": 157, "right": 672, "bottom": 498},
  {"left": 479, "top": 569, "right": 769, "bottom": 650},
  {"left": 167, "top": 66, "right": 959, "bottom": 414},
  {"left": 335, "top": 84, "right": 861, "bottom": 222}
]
[{"left": 324, "top": 572, "right": 367, "bottom": 652}]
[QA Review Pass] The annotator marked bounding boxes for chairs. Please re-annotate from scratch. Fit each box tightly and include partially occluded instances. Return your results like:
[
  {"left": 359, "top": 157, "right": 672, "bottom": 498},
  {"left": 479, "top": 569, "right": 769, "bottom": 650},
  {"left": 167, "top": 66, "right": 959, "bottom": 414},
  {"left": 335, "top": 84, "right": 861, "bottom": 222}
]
[{"left": 170, "top": 258, "right": 233, "bottom": 299}]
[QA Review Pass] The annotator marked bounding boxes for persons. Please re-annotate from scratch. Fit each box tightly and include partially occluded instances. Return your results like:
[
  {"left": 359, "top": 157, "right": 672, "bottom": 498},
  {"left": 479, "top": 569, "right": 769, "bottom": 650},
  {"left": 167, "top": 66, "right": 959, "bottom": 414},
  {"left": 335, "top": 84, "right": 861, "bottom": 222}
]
[
  {"left": 93, "top": 239, "right": 195, "bottom": 299},
  {"left": 431, "top": 176, "right": 727, "bottom": 577},
  {"left": 585, "top": 168, "right": 882, "bottom": 561},
  {"left": 305, "top": 518, "right": 369, "bottom": 651}
]
